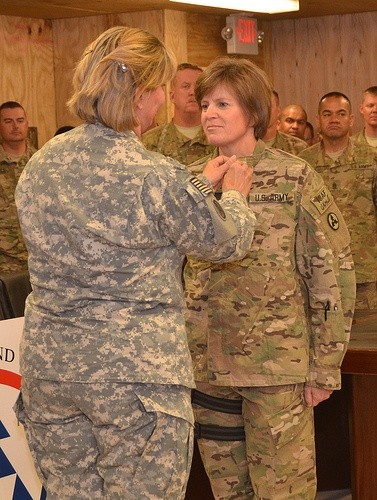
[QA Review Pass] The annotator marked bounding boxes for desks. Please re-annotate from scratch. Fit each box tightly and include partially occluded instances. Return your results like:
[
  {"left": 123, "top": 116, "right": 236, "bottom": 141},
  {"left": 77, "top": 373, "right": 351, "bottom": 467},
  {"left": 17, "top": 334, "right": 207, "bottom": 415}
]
[{"left": 340, "top": 308, "right": 377, "bottom": 500}]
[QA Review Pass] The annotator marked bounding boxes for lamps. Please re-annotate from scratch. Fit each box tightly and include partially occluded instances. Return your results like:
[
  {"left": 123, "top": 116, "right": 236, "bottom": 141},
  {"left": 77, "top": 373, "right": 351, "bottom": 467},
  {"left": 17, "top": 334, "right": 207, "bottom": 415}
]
[{"left": 221, "top": 14, "right": 264, "bottom": 55}]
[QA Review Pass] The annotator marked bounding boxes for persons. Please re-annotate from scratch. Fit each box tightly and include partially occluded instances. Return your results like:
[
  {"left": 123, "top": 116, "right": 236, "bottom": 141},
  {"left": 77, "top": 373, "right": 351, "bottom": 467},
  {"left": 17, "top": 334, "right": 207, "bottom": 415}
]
[
  {"left": 263, "top": 86, "right": 377, "bottom": 309},
  {"left": 184, "top": 57, "right": 356, "bottom": 500},
  {"left": 0, "top": 101, "right": 39, "bottom": 272},
  {"left": 13, "top": 25, "right": 255, "bottom": 500},
  {"left": 141, "top": 63, "right": 215, "bottom": 164}
]
